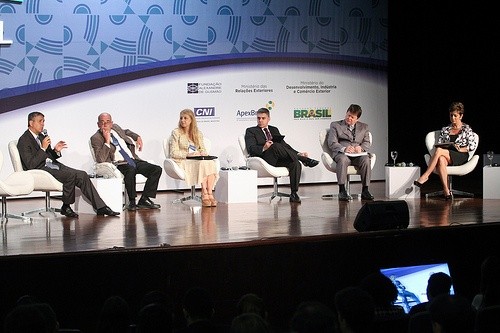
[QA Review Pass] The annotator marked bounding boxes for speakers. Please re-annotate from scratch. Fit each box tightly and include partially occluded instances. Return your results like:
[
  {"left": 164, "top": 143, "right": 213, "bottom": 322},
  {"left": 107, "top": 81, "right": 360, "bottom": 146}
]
[{"left": 352, "top": 199, "right": 409, "bottom": 232}]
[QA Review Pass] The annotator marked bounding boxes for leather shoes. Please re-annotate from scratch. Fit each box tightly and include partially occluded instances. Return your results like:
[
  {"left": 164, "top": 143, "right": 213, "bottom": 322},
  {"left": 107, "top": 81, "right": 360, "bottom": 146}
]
[
  {"left": 61, "top": 206, "right": 79, "bottom": 218},
  {"left": 128, "top": 201, "right": 136, "bottom": 211},
  {"left": 96, "top": 206, "right": 120, "bottom": 216},
  {"left": 138, "top": 198, "right": 161, "bottom": 209},
  {"left": 361, "top": 191, "right": 374, "bottom": 200},
  {"left": 303, "top": 158, "right": 320, "bottom": 168},
  {"left": 290, "top": 193, "right": 301, "bottom": 203},
  {"left": 338, "top": 191, "right": 353, "bottom": 200}
]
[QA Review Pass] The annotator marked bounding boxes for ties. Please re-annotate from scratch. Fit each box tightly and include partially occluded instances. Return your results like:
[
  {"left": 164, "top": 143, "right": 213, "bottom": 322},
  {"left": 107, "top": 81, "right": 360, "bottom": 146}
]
[
  {"left": 36, "top": 137, "right": 60, "bottom": 170},
  {"left": 263, "top": 128, "right": 272, "bottom": 141},
  {"left": 110, "top": 133, "right": 134, "bottom": 165}
]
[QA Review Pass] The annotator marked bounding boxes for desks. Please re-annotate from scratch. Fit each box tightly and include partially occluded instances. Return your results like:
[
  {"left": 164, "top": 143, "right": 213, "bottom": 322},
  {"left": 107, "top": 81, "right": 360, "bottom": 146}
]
[
  {"left": 215, "top": 170, "right": 258, "bottom": 204},
  {"left": 483, "top": 165, "right": 500, "bottom": 199},
  {"left": 74, "top": 178, "right": 122, "bottom": 214},
  {"left": 384, "top": 166, "right": 421, "bottom": 199}
]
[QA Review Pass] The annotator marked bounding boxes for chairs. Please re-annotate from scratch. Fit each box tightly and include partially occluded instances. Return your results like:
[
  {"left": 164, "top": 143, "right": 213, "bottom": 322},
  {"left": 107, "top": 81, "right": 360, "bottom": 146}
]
[
  {"left": 319, "top": 128, "right": 376, "bottom": 200},
  {"left": 8, "top": 140, "right": 64, "bottom": 218},
  {"left": 89, "top": 135, "right": 149, "bottom": 210},
  {"left": 0, "top": 149, "right": 34, "bottom": 230},
  {"left": 163, "top": 135, "right": 220, "bottom": 204},
  {"left": 424, "top": 130, "right": 479, "bottom": 200},
  {"left": 238, "top": 136, "right": 290, "bottom": 203}
]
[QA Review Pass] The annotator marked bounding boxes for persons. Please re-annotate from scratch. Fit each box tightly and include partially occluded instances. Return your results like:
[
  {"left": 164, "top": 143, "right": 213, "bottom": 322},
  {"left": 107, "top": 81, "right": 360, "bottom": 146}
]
[
  {"left": 245, "top": 108, "right": 319, "bottom": 203},
  {"left": 171, "top": 109, "right": 220, "bottom": 207},
  {"left": 0, "top": 271, "right": 500, "bottom": 333},
  {"left": 17, "top": 112, "right": 120, "bottom": 218},
  {"left": 414, "top": 103, "right": 476, "bottom": 199},
  {"left": 90, "top": 112, "right": 162, "bottom": 210},
  {"left": 328, "top": 104, "right": 372, "bottom": 199}
]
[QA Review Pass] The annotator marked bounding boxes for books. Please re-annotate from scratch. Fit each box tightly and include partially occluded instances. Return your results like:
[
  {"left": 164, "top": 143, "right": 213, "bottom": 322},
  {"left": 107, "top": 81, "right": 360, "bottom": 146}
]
[
  {"left": 345, "top": 151, "right": 367, "bottom": 157},
  {"left": 270, "top": 135, "right": 285, "bottom": 143},
  {"left": 185, "top": 156, "right": 217, "bottom": 160},
  {"left": 433, "top": 142, "right": 458, "bottom": 152}
]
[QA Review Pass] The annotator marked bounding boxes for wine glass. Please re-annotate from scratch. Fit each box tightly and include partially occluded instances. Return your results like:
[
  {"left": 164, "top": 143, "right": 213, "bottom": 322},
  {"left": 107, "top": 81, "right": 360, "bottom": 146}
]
[
  {"left": 391, "top": 151, "right": 397, "bottom": 166},
  {"left": 226, "top": 157, "right": 232, "bottom": 170},
  {"left": 487, "top": 151, "right": 494, "bottom": 166}
]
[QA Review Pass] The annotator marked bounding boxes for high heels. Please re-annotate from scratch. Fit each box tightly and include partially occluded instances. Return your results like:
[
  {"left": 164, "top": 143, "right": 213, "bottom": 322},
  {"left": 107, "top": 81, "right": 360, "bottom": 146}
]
[
  {"left": 211, "top": 195, "right": 217, "bottom": 206},
  {"left": 414, "top": 179, "right": 428, "bottom": 186},
  {"left": 445, "top": 193, "right": 452, "bottom": 202},
  {"left": 201, "top": 194, "right": 211, "bottom": 206}
]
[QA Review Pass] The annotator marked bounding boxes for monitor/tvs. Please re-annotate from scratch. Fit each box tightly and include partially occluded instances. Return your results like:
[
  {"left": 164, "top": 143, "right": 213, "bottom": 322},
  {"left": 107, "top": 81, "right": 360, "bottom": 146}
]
[{"left": 379, "top": 261, "right": 456, "bottom": 314}]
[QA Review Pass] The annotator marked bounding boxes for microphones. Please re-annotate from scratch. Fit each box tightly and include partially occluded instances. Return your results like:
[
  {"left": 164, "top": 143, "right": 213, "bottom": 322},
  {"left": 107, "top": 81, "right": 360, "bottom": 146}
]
[{"left": 43, "top": 129, "right": 50, "bottom": 149}]
